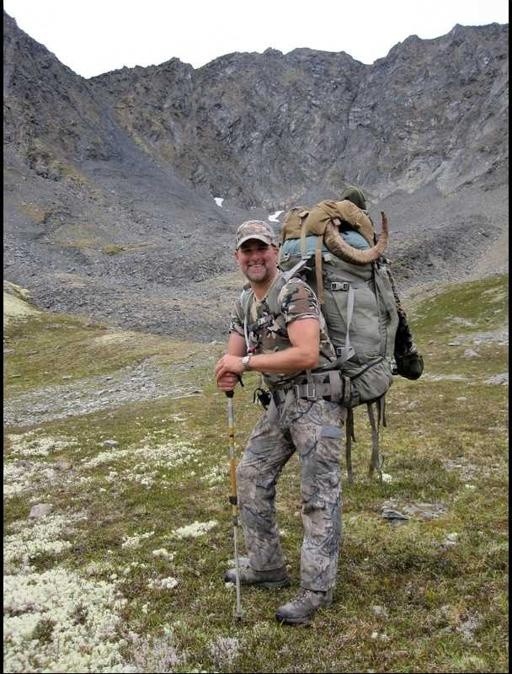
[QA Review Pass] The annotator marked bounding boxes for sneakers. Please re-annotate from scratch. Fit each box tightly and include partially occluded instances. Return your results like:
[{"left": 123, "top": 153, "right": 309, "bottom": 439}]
[
  {"left": 224, "top": 565, "right": 288, "bottom": 588},
  {"left": 274, "top": 587, "right": 333, "bottom": 625}
]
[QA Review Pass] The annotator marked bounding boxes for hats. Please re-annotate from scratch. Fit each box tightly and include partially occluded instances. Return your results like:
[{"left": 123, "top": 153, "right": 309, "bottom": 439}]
[{"left": 235, "top": 220, "right": 277, "bottom": 250}]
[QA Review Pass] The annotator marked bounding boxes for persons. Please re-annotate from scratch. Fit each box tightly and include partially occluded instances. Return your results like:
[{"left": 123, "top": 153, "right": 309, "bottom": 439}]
[{"left": 213, "top": 217, "right": 346, "bottom": 631}]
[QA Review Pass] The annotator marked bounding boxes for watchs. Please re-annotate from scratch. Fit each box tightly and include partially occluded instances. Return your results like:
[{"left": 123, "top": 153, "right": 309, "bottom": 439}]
[{"left": 242, "top": 354, "right": 253, "bottom": 371}]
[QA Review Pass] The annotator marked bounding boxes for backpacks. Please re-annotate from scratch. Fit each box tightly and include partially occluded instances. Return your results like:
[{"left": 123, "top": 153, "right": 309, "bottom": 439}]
[{"left": 240, "top": 231, "right": 399, "bottom": 410}]
